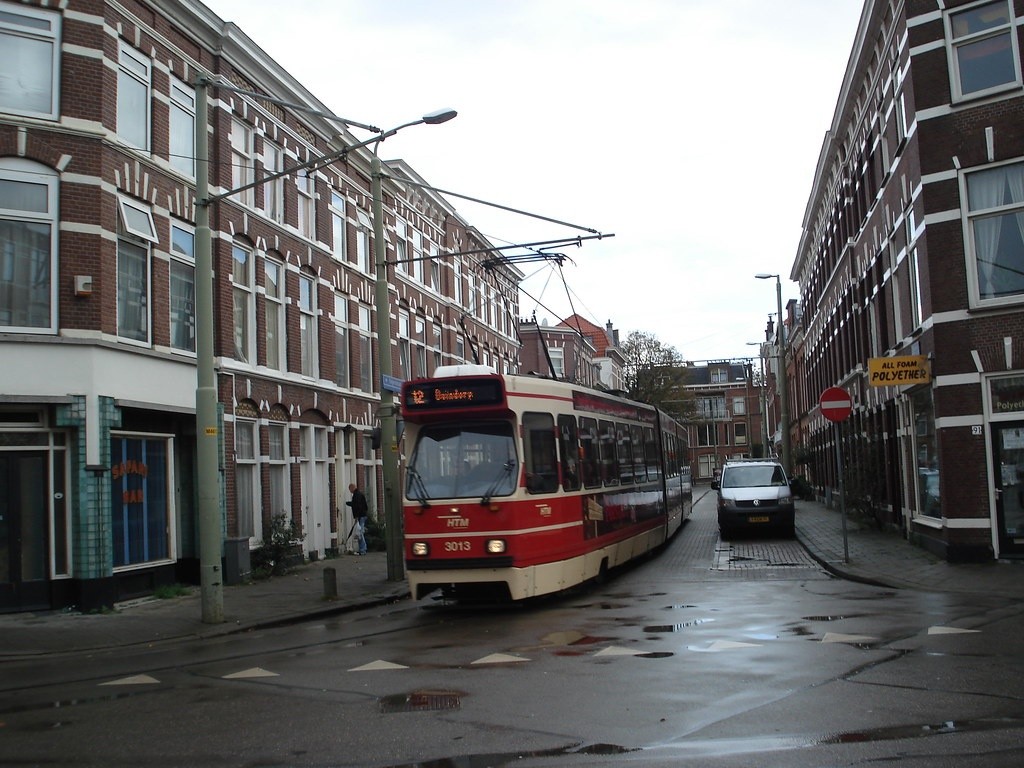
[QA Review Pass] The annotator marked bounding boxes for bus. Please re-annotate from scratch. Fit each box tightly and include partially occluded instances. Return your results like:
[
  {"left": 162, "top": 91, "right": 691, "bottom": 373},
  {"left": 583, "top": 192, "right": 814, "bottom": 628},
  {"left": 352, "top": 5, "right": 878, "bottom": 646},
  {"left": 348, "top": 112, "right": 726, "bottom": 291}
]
[{"left": 397, "top": 364, "right": 694, "bottom": 611}]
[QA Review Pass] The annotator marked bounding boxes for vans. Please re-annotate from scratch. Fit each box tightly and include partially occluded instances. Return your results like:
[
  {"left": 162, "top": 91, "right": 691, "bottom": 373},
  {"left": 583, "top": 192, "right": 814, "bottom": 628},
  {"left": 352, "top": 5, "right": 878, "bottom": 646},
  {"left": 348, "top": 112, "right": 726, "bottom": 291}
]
[{"left": 711, "top": 455, "right": 801, "bottom": 541}]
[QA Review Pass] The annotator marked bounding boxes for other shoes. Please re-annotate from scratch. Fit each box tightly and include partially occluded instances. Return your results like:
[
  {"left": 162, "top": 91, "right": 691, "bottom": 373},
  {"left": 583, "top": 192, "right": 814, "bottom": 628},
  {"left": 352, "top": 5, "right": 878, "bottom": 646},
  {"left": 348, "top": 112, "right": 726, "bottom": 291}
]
[{"left": 353, "top": 551, "right": 366, "bottom": 555}]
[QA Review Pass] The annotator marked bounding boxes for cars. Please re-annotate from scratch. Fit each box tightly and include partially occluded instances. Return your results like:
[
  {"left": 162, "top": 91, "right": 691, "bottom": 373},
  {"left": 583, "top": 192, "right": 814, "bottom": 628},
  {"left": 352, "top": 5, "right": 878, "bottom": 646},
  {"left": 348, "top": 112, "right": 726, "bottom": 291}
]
[{"left": 919, "top": 467, "right": 940, "bottom": 514}]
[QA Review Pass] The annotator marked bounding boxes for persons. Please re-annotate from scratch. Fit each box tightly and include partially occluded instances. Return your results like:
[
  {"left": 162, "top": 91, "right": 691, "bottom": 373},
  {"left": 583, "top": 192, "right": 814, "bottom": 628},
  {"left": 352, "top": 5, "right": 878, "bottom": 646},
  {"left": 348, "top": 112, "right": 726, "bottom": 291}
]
[{"left": 346, "top": 483, "right": 369, "bottom": 556}]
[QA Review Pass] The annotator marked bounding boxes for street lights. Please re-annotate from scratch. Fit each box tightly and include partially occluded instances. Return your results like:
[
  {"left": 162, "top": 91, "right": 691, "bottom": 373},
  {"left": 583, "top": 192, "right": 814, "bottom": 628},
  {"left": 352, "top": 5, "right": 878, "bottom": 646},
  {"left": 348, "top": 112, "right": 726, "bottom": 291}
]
[
  {"left": 187, "top": 72, "right": 459, "bottom": 626},
  {"left": 746, "top": 274, "right": 795, "bottom": 498}
]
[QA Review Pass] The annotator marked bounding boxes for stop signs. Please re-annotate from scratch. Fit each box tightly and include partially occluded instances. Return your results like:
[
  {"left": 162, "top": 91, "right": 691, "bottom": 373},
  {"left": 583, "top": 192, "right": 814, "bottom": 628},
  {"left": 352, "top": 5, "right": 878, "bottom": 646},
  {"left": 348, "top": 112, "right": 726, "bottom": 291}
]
[{"left": 819, "top": 387, "right": 852, "bottom": 422}]
